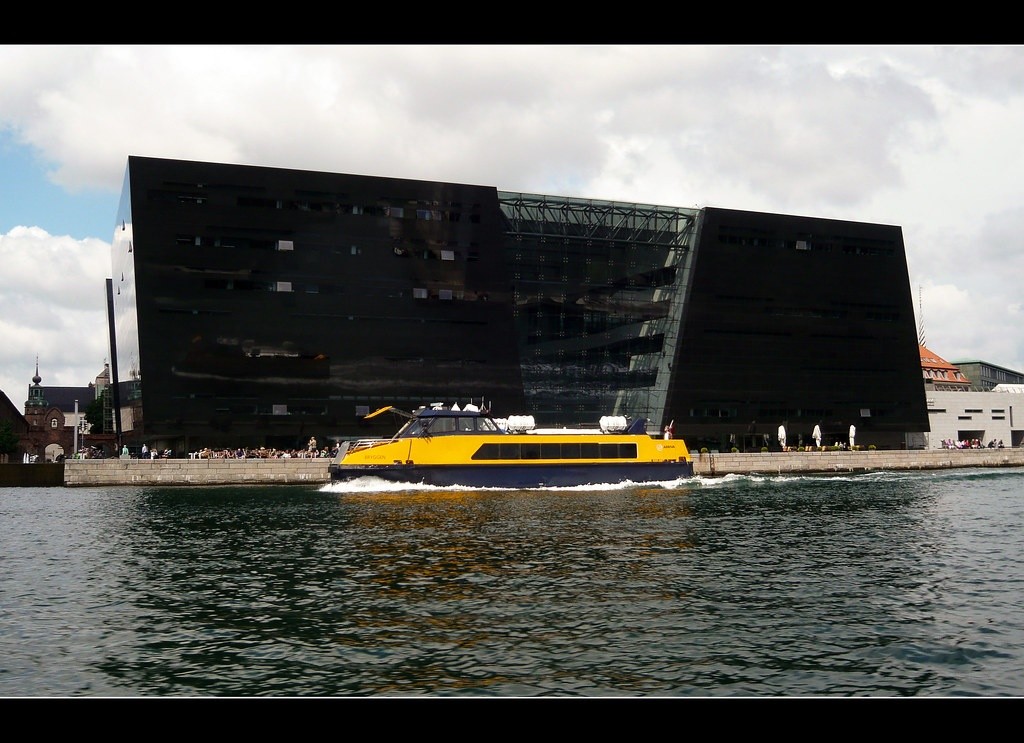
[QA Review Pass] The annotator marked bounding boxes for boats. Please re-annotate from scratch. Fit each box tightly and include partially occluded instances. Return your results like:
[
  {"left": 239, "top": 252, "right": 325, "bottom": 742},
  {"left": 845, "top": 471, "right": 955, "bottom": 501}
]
[{"left": 326, "top": 398, "right": 695, "bottom": 488}]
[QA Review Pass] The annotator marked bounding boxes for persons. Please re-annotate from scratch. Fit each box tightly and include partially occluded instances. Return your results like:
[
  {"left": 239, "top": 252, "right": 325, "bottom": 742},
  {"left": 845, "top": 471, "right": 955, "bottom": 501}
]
[
  {"left": 940, "top": 437, "right": 1005, "bottom": 449},
  {"left": 664, "top": 425, "right": 670, "bottom": 440},
  {"left": 164, "top": 448, "right": 172, "bottom": 458},
  {"left": 308, "top": 437, "right": 317, "bottom": 459},
  {"left": 199, "top": 446, "right": 341, "bottom": 460},
  {"left": 121, "top": 445, "right": 129, "bottom": 456},
  {"left": 141, "top": 444, "right": 148, "bottom": 459}
]
